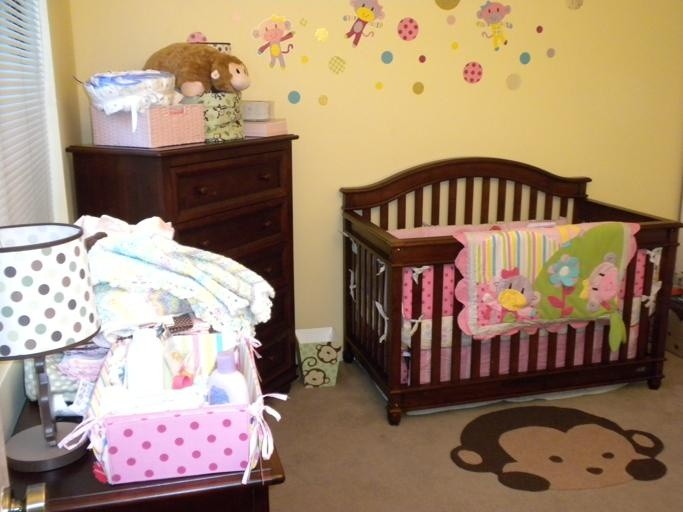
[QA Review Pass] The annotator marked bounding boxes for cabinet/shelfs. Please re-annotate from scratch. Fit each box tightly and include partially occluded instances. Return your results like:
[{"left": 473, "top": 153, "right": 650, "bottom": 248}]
[{"left": 66, "top": 133, "right": 297, "bottom": 399}]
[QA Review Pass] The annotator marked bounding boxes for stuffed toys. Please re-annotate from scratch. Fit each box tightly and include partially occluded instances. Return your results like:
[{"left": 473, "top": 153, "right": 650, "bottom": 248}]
[{"left": 139, "top": 43, "right": 250, "bottom": 99}]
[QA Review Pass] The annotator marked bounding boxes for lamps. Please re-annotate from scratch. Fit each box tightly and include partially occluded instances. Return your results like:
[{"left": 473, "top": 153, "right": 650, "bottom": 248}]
[{"left": 0, "top": 224, "right": 101, "bottom": 473}]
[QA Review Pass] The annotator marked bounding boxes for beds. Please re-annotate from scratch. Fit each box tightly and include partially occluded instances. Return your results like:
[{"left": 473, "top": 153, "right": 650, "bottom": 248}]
[{"left": 340, "top": 157, "right": 683, "bottom": 424}]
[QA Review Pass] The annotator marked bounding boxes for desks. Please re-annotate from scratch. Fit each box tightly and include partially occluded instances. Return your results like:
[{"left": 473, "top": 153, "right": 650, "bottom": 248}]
[{"left": 1, "top": 392, "right": 285, "bottom": 512}]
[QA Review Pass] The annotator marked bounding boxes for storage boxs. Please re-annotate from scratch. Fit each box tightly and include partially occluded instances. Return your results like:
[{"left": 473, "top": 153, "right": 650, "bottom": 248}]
[{"left": 57, "top": 332, "right": 291, "bottom": 486}]
